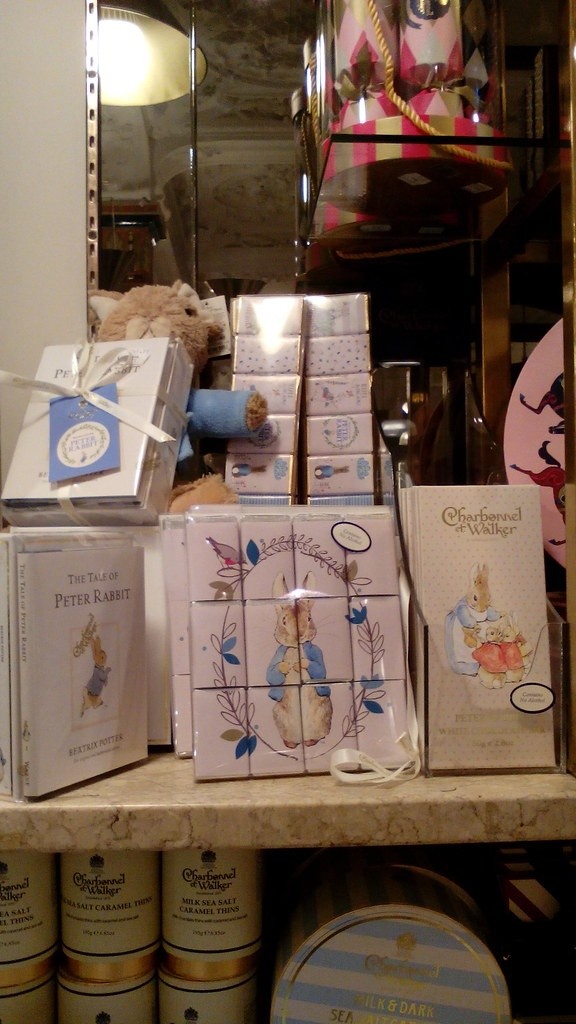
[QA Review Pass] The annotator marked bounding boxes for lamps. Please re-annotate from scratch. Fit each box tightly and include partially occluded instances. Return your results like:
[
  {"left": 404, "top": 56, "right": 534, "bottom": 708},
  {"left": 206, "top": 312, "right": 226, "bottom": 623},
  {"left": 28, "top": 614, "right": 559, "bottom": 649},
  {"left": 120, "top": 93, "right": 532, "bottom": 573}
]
[{"left": 97, "top": 1, "right": 208, "bottom": 106}]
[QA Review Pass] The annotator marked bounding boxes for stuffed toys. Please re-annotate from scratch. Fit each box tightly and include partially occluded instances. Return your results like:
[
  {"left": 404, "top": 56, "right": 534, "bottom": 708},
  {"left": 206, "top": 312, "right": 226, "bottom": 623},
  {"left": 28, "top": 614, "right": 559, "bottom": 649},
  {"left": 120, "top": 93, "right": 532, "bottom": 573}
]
[{"left": 86, "top": 279, "right": 268, "bottom": 516}]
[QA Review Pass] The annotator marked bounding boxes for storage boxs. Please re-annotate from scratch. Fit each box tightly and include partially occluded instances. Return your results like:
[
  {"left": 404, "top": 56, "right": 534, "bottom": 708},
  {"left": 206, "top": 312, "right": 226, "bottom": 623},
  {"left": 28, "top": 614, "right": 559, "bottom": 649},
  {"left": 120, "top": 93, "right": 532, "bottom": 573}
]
[{"left": 2, "top": 336, "right": 196, "bottom": 526}]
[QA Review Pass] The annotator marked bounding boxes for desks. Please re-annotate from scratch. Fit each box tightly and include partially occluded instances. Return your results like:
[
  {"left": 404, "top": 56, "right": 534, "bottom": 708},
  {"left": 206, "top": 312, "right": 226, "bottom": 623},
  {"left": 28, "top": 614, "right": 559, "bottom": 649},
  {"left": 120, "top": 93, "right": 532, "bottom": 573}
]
[{"left": 0, "top": 751, "right": 576, "bottom": 846}]
